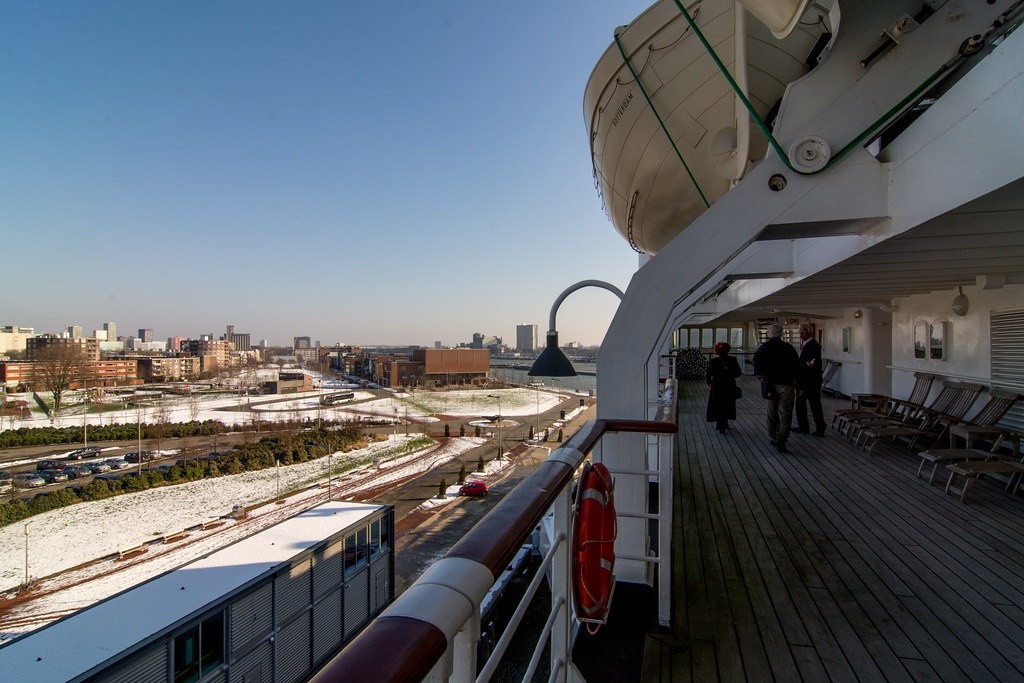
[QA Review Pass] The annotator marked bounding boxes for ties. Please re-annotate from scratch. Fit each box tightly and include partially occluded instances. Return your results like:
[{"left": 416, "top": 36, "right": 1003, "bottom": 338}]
[{"left": 800, "top": 344, "right": 803, "bottom": 355}]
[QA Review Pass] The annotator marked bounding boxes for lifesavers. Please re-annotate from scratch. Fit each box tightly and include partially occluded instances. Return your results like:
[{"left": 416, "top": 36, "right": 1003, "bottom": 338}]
[{"left": 575, "top": 461, "right": 617, "bottom": 627}]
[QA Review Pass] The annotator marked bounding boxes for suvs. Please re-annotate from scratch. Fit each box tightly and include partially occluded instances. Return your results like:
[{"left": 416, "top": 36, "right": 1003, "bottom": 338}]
[{"left": 68, "top": 446, "right": 102, "bottom": 460}]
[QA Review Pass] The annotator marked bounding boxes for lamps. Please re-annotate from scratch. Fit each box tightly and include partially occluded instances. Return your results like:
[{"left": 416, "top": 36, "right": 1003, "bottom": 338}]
[
  {"left": 527, "top": 279, "right": 625, "bottom": 377},
  {"left": 952, "top": 294, "right": 969, "bottom": 316}
]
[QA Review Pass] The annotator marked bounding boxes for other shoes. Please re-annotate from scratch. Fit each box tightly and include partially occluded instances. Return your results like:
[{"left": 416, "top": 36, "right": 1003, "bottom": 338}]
[
  {"left": 769, "top": 435, "right": 776, "bottom": 443},
  {"left": 812, "top": 432, "right": 825, "bottom": 437},
  {"left": 776, "top": 443, "right": 785, "bottom": 451},
  {"left": 792, "top": 427, "right": 809, "bottom": 433}
]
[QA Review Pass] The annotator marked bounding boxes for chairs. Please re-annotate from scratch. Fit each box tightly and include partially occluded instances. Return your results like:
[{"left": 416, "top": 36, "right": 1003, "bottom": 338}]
[{"left": 820, "top": 358, "right": 1024, "bottom": 503}]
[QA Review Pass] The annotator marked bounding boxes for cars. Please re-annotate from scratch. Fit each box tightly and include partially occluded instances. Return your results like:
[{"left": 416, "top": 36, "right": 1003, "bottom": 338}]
[
  {"left": 459, "top": 482, "right": 488, "bottom": 497},
  {"left": 92, "top": 461, "right": 111, "bottom": 472},
  {"left": 138, "top": 451, "right": 147, "bottom": 457},
  {"left": 0, "top": 480, "right": 15, "bottom": 494},
  {"left": 124, "top": 453, "right": 145, "bottom": 463},
  {"left": 84, "top": 462, "right": 103, "bottom": 473},
  {"left": 39, "top": 470, "right": 68, "bottom": 483},
  {"left": 62, "top": 465, "right": 91, "bottom": 478},
  {"left": 146, "top": 451, "right": 158, "bottom": 459},
  {"left": 100, "top": 458, "right": 129, "bottom": 469},
  {"left": 0, "top": 471, "right": 14, "bottom": 484},
  {"left": 13, "top": 474, "right": 45, "bottom": 488}
]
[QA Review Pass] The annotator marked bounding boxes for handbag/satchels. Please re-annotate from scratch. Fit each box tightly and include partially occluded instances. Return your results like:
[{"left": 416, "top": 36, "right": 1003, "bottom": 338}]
[
  {"left": 760, "top": 377, "right": 775, "bottom": 401},
  {"left": 735, "top": 386, "right": 742, "bottom": 400}
]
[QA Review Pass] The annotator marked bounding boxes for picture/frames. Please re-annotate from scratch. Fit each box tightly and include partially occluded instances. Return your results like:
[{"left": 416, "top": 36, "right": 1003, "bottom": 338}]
[
  {"left": 911, "top": 320, "right": 947, "bottom": 362},
  {"left": 842, "top": 326, "right": 851, "bottom": 353}
]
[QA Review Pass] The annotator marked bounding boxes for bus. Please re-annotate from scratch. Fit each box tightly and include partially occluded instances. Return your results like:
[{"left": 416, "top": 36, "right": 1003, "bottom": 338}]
[{"left": 325, "top": 393, "right": 354, "bottom": 405}]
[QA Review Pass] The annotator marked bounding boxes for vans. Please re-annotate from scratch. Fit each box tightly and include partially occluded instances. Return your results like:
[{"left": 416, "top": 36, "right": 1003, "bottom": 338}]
[{"left": 37, "top": 460, "right": 66, "bottom": 471}]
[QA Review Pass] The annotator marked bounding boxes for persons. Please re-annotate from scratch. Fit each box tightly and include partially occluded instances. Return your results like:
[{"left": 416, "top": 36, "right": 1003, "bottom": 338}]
[
  {"left": 705, "top": 342, "right": 742, "bottom": 435},
  {"left": 791, "top": 322, "right": 826, "bottom": 436},
  {"left": 752, "top": 324, "right": 802, "bottom": 453}
]
